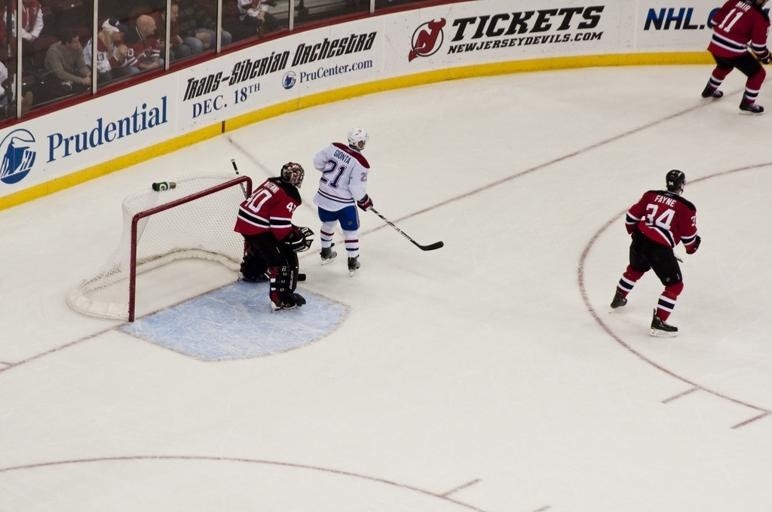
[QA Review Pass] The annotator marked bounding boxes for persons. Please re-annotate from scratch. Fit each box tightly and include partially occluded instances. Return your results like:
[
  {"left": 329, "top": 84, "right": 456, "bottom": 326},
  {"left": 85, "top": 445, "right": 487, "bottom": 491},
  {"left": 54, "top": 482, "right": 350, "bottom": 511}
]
[
  {"left": 0, "top": 0, "right": 281, "bottom": 119},
  {"left": 312, "top": 127, "right": 373, "bottom": 271},
  {"left": 610, "top": 170, "right": 702, "bottom": 332},
  {"left": 700, "top": 0, "right": 772, "bottom": 113},
  {"left": 232, "top": 161, "right": 314, "bottom": 311}
]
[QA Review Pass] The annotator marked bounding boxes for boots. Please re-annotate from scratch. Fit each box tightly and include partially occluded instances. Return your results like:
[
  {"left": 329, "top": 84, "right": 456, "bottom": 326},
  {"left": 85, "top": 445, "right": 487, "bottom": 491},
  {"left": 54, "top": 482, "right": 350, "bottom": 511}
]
[
  {"left": 321, "top": 246, "right": 337, "bottom": 261},
  {"left": 651, "top": 315, "right": 677, "bottom": 332},
  {"left": 702, "top": 81, "right": 723, "bottom": 98},
  {"left": 739, "top": 94, "right": 764, "bottom": 113},
  {"left": 347, "top": 255, "right": 360, "bottom": 270},
  {"left": 610, "top": 291, "right": 627, "bottom": 308}
]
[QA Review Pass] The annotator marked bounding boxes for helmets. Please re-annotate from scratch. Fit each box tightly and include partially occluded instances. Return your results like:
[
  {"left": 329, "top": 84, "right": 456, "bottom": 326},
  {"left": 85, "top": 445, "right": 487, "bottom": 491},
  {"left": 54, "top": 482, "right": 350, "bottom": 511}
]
[
  {"left": 281, "top": 162, "right": 304, "bottom": 187},
  {"left": 347, "top": 127, "right": 368, "bottom": 150},
  {"left": 666, "top": 169, "right": 685, "bottom": 192}
]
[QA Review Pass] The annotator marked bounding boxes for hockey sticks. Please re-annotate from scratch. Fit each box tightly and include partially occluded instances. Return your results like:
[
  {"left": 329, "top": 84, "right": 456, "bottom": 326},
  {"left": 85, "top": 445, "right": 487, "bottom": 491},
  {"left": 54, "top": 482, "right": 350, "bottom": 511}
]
[
  {"left": 369, "top": 204, "right": 443, "bottom": 250},
  {"left": 232, "top": 160, "right": 306, "bottom": 281}
]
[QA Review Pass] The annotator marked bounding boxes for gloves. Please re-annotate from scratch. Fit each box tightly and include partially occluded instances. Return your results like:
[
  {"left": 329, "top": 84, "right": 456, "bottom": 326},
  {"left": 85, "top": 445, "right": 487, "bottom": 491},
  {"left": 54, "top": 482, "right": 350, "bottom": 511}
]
[
  {"left": 357, "top": 194, "right": 373, "bottom": 211},
  {"left": 285, "top": 231, "right": 307, "bottom": 252},
  {"left": 694, "top": 235, "right": 701, "bottom": 248},
  {"left": 762, "top": 53, "right": 772, "bottom": 64}
]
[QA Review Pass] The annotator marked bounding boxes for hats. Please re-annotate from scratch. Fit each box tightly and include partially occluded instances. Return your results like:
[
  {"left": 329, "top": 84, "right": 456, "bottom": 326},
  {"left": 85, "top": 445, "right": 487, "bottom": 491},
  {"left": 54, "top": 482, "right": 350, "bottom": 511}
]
[{"left": 101, "top": 17, "right": 128, "bottom": 32}]
